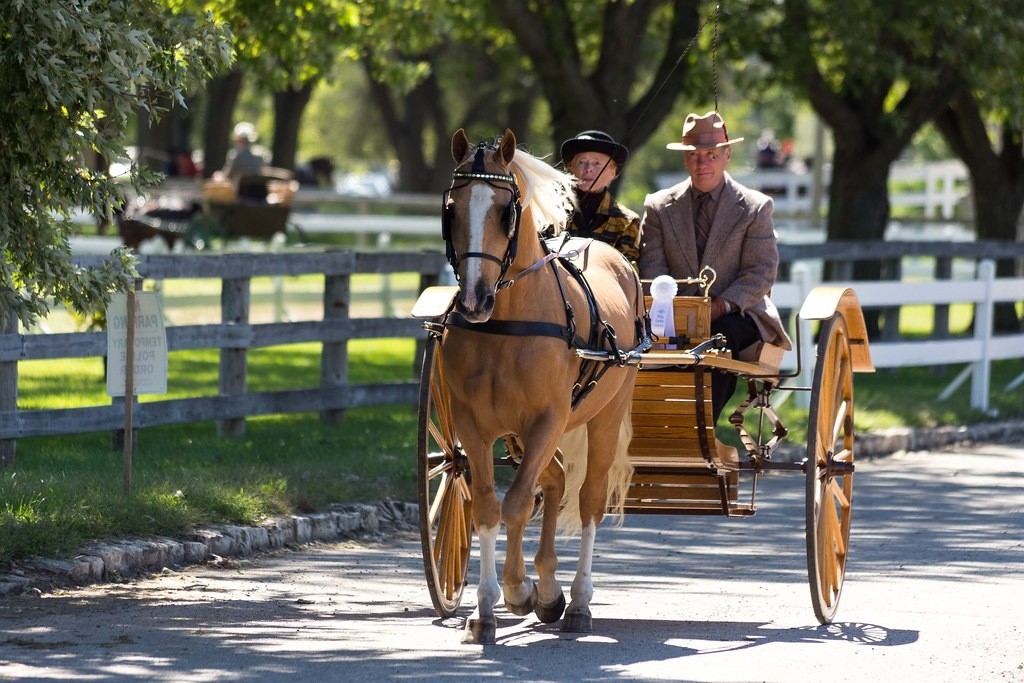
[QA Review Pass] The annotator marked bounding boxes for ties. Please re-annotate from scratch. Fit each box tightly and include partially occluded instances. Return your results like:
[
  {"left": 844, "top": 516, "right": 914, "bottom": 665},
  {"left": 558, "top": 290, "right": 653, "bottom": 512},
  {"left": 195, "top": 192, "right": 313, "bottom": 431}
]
[{"left": 694, "top": 193, "right": 712, "bottom": 261}]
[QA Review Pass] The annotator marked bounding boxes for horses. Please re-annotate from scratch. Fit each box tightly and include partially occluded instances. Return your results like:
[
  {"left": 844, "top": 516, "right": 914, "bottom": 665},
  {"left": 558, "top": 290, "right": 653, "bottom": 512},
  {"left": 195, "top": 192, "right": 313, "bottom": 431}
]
[{"left": 444, "top": 126, "right": 644, "bottom": 641}]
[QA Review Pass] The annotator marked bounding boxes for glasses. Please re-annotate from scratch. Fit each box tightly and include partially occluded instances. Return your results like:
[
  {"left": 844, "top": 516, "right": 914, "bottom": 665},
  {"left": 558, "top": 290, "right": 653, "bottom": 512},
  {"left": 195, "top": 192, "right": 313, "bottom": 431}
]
[{"left": 686, "top": 149, "right": 718, "bottom": 160}]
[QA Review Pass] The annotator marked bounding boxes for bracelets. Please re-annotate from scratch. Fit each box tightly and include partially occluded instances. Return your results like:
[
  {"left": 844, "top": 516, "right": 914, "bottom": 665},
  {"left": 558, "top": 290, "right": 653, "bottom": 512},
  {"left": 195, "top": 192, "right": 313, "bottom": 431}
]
[{"left": 724, "top": 300, "right": 731, "bottom": 314}]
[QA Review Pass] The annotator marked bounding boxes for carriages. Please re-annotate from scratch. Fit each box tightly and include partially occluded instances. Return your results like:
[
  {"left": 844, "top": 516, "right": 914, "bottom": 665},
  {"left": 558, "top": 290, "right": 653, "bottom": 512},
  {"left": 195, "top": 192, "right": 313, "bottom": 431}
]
[{"left": 405, "top": 126, "right": 876, "bottom": 645}]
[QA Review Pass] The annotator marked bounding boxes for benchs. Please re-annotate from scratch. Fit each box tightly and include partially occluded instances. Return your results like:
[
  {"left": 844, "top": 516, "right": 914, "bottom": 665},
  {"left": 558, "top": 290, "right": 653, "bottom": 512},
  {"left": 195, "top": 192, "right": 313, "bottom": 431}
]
[{"left": 205, "top": 168, "right": 299, "bottom": 213}]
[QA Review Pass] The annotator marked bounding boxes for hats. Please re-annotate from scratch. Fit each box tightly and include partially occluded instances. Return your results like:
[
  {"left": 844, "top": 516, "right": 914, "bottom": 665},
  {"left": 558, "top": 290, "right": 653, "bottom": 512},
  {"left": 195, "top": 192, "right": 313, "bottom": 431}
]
[
  {"left": 666, "top": 111, "right": 744, "bottom": 150},
  {"left": 560, "top": 130, "right": 629, "bottom": 180}
]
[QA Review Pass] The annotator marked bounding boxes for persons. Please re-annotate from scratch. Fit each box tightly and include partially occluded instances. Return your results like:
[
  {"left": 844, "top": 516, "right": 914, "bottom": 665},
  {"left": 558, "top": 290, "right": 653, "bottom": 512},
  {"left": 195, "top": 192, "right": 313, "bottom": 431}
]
[
  {"left": 755, "top": 138, "right": 784, "bottom": 196},
  {"left": 213, "top": 121, "right": 273, "bottom": 184},
  {"left": 636, "top": 112, "right": 793, "bottom": 431},
  {"left": 538, "top": 130, "right": 639, "bottom": 270}
]
